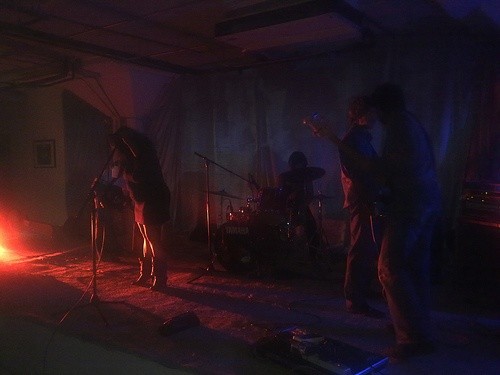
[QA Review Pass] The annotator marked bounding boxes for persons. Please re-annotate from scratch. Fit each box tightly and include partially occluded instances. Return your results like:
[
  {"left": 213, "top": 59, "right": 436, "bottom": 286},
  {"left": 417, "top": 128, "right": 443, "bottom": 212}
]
[
  {"left": 339, "top": 95, "right": 384, "bottom": 311},
  {"left": 277, "top": 151, "right": 323, "bottom": 247},
  {"left": 108, "top": 127, "right": 170, "bottom": 291},
  {"left": 370, "top": 84, "right": 441, "bottom": 357}
]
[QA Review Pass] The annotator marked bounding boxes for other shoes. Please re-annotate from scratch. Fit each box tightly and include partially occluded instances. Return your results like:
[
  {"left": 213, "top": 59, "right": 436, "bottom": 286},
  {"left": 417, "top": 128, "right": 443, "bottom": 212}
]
[{"left": 381, "top": 340, "right": 423, "bottom": 357}]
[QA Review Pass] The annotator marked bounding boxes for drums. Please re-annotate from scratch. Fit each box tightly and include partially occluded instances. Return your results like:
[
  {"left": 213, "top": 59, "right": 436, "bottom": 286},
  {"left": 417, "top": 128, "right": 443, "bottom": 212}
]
[{"left": 279, "top": 207, "right": 313, "bottom": 239}]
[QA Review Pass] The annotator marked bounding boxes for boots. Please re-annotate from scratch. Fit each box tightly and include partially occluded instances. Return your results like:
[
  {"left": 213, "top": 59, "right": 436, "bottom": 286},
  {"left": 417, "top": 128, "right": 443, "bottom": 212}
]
[
  {"left": 130, "top": 256, "right": 154, "bottom": 286},
  {"left": 149, "top": 255, "right": 168, "bottom": 290}
]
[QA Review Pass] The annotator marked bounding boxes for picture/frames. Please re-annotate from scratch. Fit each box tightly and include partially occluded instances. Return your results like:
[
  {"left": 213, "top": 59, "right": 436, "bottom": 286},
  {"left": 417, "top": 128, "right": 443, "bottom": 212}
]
[{"left": 33, "top": 139, "right": 56, "bottom": 168}]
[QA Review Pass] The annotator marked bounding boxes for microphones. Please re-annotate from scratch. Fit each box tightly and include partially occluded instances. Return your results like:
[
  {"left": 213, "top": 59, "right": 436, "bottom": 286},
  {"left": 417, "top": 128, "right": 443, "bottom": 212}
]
[
  {"left": 248, "top": 173, "right": 260, "bottom": 189},
  {"left": 303, "top": 118, "right": 321, "bottom": 137}
]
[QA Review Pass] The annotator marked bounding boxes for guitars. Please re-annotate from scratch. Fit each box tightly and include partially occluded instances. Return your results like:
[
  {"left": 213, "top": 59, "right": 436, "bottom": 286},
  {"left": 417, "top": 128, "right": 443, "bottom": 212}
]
[{"left": 302, "top": 116, "right": 373, "bottom": 180}]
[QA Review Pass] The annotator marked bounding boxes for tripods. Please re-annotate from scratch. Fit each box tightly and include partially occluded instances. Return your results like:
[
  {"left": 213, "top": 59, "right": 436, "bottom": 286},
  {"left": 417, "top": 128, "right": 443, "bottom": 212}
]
[
  {"left": 186, "top": 152, "right": 253, "bottom": 283},
  {"left": 305, "top": 199, "right": 328, "bottom": 249},
  {"left": 64, "top": 156, "right": 131, "bottom": 325}
]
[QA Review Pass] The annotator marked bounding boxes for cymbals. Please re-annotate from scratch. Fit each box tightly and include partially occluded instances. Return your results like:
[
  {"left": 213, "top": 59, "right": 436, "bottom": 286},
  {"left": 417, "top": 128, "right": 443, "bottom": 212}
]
[
  {"left": 202, "top": 189, "right": 244, "bottom": 200},
  {"left": 275, "top": 166, "right": 326, "bottom": 183}
]
